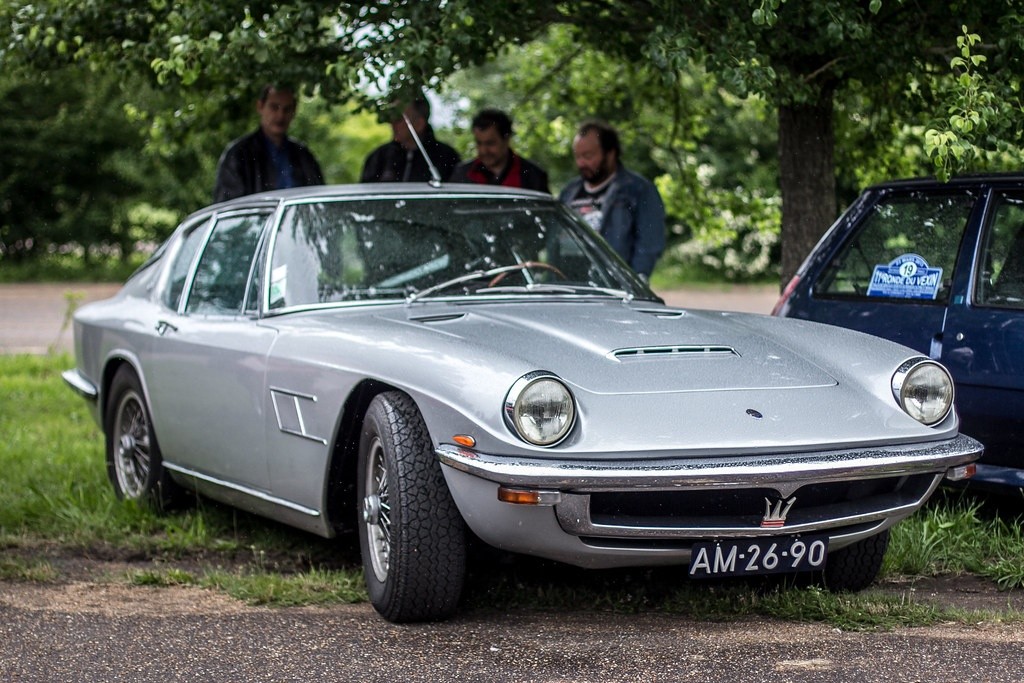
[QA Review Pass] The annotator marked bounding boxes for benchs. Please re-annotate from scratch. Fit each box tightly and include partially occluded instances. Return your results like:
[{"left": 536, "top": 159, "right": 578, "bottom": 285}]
[{"left": 313, "top": 218, "right": 481, "bottom": 289}]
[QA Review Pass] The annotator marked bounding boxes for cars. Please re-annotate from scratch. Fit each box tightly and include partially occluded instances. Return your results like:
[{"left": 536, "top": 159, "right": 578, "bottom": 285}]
[
  {"left": 59, "top": 181, "right": 985, "bottom": 622},
  {"left": 768, "top": 173, "right": 1024, "bottom": 489}
]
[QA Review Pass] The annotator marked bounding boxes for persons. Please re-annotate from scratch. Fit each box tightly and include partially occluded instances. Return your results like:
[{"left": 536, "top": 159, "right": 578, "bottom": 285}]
[
  {"left": 212, "top": 77, "right": 342, "bottom": 296},
  {"left": 547, "top": 121, "right": 666, "bottom": 292},
  {"left": 354, "top": 86, "right": 466, "bottom": 299},
  {"left": 449, "top": 104, "right": 555, "bottom": 289}
]
